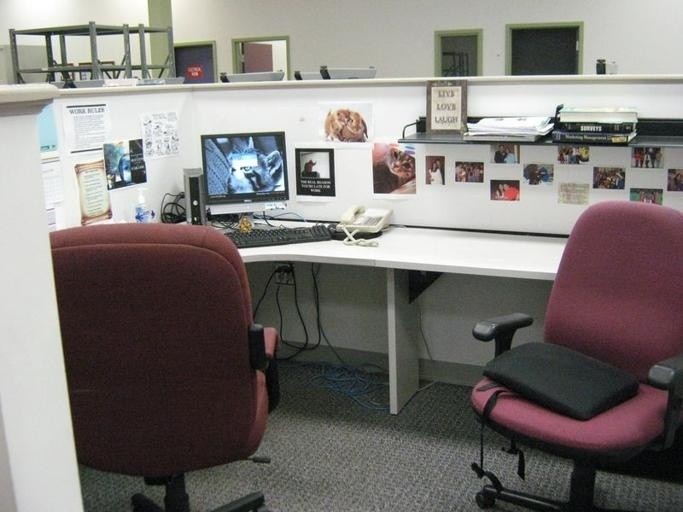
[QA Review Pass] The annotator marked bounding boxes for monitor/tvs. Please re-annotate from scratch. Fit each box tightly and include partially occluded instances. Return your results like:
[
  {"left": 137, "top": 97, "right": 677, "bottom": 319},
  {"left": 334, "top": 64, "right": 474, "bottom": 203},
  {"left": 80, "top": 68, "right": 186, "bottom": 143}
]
[{"left": 201, "top": 131, "right": 290, "bottom": 229}]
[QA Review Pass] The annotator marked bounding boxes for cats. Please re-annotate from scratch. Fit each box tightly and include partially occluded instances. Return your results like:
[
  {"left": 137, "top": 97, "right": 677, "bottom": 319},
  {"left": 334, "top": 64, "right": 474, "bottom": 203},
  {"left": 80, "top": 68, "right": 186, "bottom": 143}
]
[
  {"left": 226, "top": 136, "right": 282, "bottom": 193},
  {"left": 372, "top": 147, "right": 416, "bottom": 194}
]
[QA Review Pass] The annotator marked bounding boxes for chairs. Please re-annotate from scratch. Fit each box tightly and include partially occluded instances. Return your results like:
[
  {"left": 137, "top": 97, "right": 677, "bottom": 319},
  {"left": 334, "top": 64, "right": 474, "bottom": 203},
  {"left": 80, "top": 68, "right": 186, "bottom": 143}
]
[
  {"left": 470, "top": 200, "right": 682, "bottom": 512},
  {"left": 48, "top": 223, "right": 279, "bottom": 512}
]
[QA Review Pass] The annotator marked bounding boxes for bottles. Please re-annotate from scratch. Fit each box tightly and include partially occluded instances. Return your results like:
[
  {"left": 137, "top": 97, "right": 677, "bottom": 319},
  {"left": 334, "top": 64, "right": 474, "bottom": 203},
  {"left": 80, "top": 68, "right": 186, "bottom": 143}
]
[
  {"left": 595, "top": 58, "right": 618, "bottom": 74},
  {"left": 134, "top": 188, "right": 156, "bottom": 224}
]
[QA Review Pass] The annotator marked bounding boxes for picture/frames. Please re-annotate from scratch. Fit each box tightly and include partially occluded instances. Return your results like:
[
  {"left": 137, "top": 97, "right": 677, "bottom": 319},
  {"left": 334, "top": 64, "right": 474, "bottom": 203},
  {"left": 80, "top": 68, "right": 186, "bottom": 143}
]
[{"left": 427, "top": 80, "right": 467, "bottom": 134}]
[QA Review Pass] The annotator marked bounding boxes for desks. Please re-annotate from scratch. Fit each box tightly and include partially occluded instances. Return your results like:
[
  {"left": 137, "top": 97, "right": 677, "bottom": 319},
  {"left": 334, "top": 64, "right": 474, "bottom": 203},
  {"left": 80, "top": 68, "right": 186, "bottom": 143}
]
[{"left": 178, "top": 217, "right": 569, "bottom": 414}]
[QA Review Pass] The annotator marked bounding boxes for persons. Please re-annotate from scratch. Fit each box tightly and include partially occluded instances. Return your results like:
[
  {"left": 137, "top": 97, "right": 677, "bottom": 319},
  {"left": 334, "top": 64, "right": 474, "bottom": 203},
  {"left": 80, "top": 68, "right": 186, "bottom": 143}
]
[
  {"left": 495, "top": 183, "right": 519, "bottom": 200},
  {"left": 456, "top": 164, "right": 483, "bottom": 182},
  {"left": 429, "top": 160, "right": 443, "bottom": 184},
  {"left": 494, "top": 145, "right": 515, "bottom": 163},
  {"left": 634, "top": 149, "right": 662, "bottom": 168}
]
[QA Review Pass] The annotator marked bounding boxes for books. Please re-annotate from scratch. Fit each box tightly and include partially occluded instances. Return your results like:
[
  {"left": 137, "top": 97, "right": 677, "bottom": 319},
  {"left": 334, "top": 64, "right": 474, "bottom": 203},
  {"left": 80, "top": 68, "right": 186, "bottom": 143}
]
[
  {"left": 462, "top": 117, "right": 555, "bottom": 143},
  {"left": 552, "top": 106, "right": 638, "bottom": 144}
]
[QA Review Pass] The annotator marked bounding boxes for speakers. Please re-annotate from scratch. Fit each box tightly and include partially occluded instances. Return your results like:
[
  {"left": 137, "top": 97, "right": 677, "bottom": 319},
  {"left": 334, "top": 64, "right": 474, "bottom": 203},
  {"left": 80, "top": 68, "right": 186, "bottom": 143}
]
[{"left": 184, "top": 168, "right": 206, "bottom": 224}]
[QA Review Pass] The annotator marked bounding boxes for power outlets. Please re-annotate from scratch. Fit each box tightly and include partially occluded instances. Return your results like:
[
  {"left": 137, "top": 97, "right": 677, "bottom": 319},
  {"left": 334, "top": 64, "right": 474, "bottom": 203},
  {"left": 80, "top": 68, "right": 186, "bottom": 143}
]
[{"left": 275, "top": 263, "right": 294, "bottom": 285}]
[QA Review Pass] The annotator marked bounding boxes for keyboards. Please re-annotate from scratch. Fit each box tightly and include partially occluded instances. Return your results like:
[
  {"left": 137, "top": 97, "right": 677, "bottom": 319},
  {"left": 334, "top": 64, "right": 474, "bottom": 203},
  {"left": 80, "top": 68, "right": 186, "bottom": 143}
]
[{"left": 225, "top": 225, "right": 331, "bottom": 248}]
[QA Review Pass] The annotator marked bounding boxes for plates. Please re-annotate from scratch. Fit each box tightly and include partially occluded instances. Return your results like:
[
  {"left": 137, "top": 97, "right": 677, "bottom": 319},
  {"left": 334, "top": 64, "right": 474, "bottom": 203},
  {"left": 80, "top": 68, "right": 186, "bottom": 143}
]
[
  {"left": 328, "top": 68, "right": 374, "bottom": 79},
  {"left": 76, "top": 77, "right": 186, "bottom": 88},
  {"left": 299, "top": 72, "right": 324, "bottom": 78},
  {"left": 227, "top": 71, "right": 284, "bottom": 82}
]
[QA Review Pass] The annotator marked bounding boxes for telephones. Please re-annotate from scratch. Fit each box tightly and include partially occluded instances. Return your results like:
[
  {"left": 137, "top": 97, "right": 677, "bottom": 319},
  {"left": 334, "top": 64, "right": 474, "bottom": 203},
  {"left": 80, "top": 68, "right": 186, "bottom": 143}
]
[{"left": 336, "top": 206, "right": 393, "bottom": 234}]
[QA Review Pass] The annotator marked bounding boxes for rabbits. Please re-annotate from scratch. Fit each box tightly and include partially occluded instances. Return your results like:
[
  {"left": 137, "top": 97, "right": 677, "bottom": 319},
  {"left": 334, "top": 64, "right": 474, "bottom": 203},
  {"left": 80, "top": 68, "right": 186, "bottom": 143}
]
[{"left": 324, "top": 109, "right": 368, "bottom": 143}]
